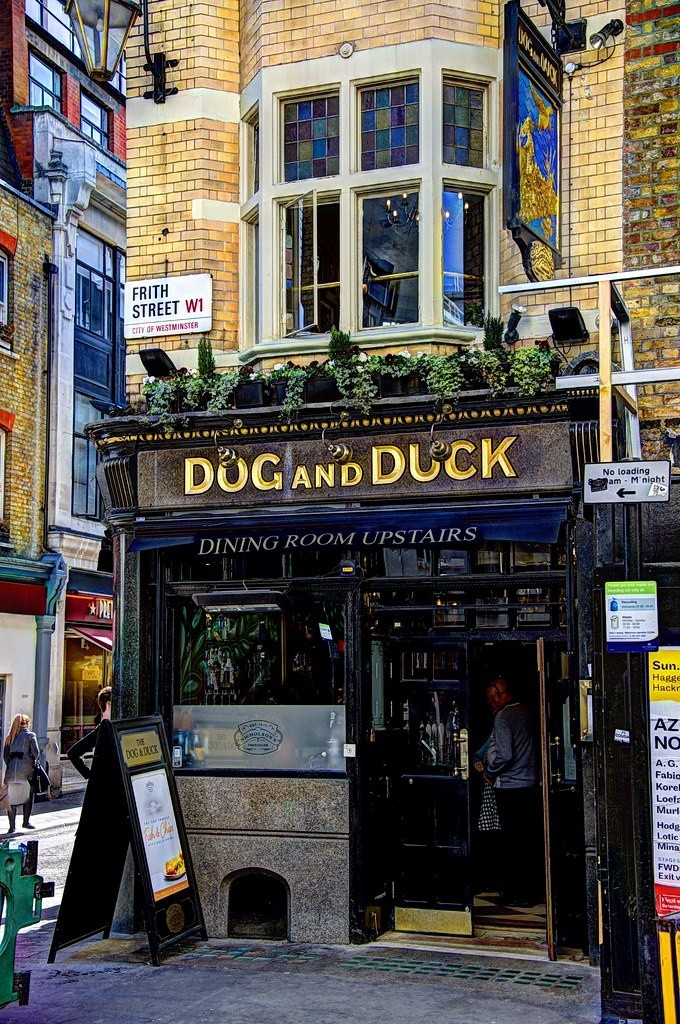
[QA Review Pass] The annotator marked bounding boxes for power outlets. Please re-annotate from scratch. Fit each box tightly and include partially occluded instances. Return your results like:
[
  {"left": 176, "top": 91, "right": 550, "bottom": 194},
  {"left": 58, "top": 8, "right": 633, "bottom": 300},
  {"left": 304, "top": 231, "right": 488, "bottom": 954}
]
[{"left": 365, "top": 905, "right": 382, "bottom": 931}]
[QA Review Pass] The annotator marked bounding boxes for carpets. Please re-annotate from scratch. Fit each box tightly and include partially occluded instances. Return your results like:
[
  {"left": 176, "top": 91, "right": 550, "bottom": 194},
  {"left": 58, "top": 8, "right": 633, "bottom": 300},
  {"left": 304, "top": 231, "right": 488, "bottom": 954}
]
[{"left": 471, "top": 892, "right": 558, "bottom": 930}]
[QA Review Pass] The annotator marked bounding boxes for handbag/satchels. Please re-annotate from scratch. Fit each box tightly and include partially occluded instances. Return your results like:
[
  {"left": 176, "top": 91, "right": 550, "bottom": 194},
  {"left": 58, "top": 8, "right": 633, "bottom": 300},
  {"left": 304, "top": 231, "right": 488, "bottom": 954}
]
[{"left": 33, "top": 761, "right": 51, "bottom": 793}]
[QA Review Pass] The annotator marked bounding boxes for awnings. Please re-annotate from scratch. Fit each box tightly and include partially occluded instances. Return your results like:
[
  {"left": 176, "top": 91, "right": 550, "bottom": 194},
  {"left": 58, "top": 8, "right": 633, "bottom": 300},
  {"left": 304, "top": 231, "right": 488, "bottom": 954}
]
[{"left": 66, "top": 625, "right": 114, "bottom": 653}]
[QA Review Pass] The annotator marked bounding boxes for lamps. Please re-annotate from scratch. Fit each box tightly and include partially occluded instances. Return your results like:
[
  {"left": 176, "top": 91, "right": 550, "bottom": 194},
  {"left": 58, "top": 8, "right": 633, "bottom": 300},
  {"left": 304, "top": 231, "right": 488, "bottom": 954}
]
[
  {"left": 213, "top": 419, "right": 243, "bottom": 468},
  {"left": 52, "top": 0, "right": 180, "bottom": 104},
  {"left": 321, "top": 410, "right": 353, "bottom": 466},
  {"left": 138, "top": 348, "right": 177, "bottom": 377},
  {"left": 385, "top": 191, "right": 470, "bottom": 236},
  {"left": 504, "top": 304, "right": 528, "bottom": 345},
  {"left": 88, "top": 399, "right": 123, "bottom": 416},
  {"left": 589, "top": 18, "right": 625, "bottom": 50},
  {"left": 548, "top": 306, "right": 589, "bottom": 344},
  {"left": 428, "top": 403, "right": 453, "bottom": 461},
  {"left": 191, "top": 591, "right": 293, "bottom": 616}
]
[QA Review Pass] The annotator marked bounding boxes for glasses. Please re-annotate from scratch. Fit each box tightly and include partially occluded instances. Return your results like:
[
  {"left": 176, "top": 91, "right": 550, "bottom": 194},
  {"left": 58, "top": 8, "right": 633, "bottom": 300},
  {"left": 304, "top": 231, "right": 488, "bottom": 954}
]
[{"left": 490, "top": 682, "right": 501, "bottom": 695}]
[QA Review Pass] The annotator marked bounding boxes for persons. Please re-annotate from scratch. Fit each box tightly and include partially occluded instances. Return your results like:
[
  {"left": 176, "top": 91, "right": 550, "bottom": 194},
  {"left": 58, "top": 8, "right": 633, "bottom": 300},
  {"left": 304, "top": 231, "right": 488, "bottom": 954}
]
[
  {"left": 3, "top": 714, "right": 40, "bottom": 833},
  {"left": 473, "top": 680, "right": 546, "bottom": 906},
  {"left": 66, "top": 687, "right": 112, "bottom": 780}
]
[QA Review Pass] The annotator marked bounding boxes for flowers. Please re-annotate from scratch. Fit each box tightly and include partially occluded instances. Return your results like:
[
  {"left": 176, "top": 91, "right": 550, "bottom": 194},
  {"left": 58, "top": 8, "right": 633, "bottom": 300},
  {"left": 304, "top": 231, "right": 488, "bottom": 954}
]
[
  {"left": 141, "top": 339, "right": 568, "bottom": 434},
  {"left": 0, "top": 321, "right": 16, "bottom": 343}
]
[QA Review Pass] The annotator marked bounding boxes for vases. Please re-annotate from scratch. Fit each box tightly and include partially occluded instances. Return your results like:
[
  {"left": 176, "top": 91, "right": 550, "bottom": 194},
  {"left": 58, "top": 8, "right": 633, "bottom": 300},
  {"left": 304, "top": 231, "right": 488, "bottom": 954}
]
[
  {"left": 416, "top": 364, "right": 442, "bottom": 394},
  {"left": 274, "top": 375, "right": 307, "bottom": 407},
  {"left": 231, "top": 377, "right": 267, "bottom": 408},
  {"left": 0, "top": 338, "right": 11, "bottom": 350},
  {"left": 381, "top": 365, "right": 409, "bottom": 397},
  {"left": 453, "top": 362, "right": 481, "bottom": 391},
  {"left": 183, "top": 388, "right": 225, "bottom": 413},
  {"left": 535, "top": 355, "right": 563, "bottom": 385},
  {"left": 497, "top": 358, "right": 525, "bottom": 387},
  {"left": 143, "top": 383, "right": 182, "bottom": 416},
  {"left": 349, "top": 373, "right": 382, "bottom": 400},
  {"left": 309, "top": 373, "right": 344, "bottom": 403}
]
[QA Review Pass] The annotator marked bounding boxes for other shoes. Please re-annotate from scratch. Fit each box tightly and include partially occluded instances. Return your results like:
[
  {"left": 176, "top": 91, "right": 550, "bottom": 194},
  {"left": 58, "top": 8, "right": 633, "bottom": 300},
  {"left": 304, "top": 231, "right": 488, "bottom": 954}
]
[
  {"left": 8, "top": 828, "right": 14, "bottom": 833},
  {"left": 22, "top": 824, "right": 34, "bottom": 829},
  {"left": 495, "top": 896, "right": 531, "bottom": 908}
]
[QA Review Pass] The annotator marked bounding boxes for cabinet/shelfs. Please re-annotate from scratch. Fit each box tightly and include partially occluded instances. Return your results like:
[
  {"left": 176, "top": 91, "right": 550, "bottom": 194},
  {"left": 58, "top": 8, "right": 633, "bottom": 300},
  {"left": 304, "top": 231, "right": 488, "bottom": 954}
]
[{"left": 391, "top": 773, "right": 475, "bottom": 939}]
